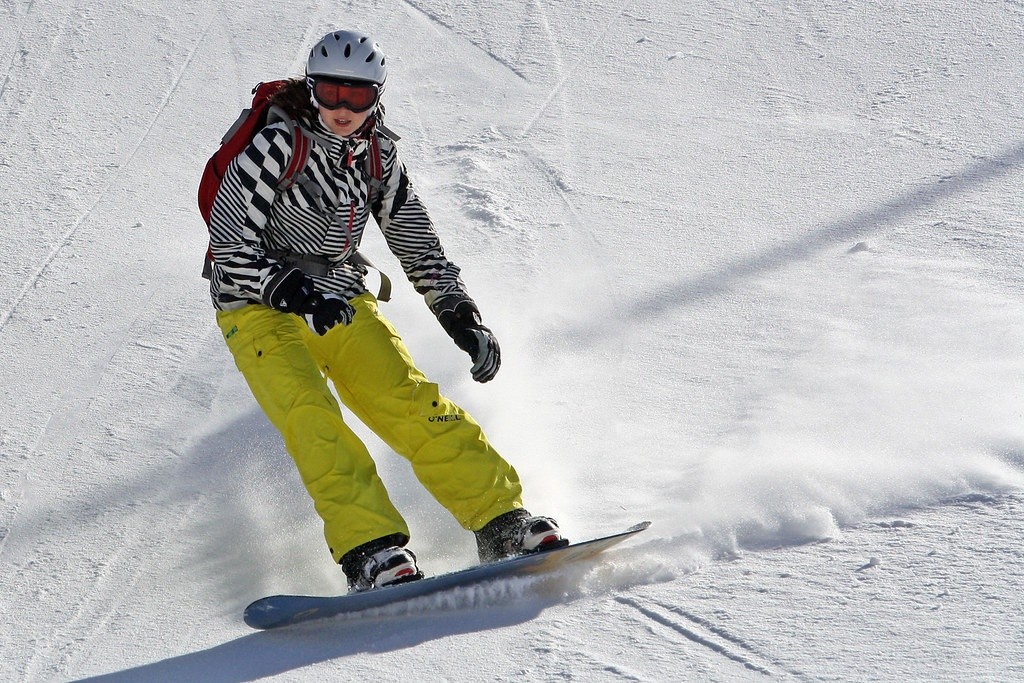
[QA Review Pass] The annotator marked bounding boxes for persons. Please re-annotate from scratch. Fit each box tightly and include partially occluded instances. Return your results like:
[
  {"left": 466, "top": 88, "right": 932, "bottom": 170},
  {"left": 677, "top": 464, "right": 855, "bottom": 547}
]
[{"left": 207, "top": 31, "right": 571, "bottom": 594}]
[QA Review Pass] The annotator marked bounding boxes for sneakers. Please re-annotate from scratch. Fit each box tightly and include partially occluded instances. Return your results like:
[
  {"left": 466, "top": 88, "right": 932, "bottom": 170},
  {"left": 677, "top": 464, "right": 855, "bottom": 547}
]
[
  {"left": 474, "top": 507, "right": 570, "bottom": 565},
  {"left": 342, "top": 544, "right": 425, "bottom": 593}
]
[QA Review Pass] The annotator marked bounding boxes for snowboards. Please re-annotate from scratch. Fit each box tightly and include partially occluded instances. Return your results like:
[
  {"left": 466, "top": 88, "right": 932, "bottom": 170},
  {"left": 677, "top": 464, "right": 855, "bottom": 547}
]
[{"left": 241, "top": 519, "right": 652, "bottom": 631}]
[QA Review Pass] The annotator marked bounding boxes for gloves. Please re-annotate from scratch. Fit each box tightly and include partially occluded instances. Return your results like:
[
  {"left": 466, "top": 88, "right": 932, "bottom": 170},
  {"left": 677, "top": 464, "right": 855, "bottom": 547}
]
[
  {"left": 262, "top": 264, "right": 357, "bottom": 335},
  {"left": 436, "top": 295, "right": 501, "bottom": 383}
]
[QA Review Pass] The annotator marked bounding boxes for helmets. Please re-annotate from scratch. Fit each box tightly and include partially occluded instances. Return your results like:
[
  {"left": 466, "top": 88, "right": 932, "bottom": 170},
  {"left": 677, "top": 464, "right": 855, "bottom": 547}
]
[{"left": 306, "top": 30, "right": 388, "bottom": 96}]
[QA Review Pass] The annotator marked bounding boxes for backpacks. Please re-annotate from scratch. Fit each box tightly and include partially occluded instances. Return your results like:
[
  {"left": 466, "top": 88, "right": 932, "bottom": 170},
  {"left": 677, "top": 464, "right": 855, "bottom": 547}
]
[{"left": 198, "top": 81, "right": 383, "bottom": 260}]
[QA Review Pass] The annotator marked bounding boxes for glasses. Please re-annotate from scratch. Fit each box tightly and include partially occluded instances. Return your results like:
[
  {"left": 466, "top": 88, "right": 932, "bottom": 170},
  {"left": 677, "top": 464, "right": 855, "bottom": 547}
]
[{"left": 311, "top": 79, "right": 378, "bottom": 114}]
